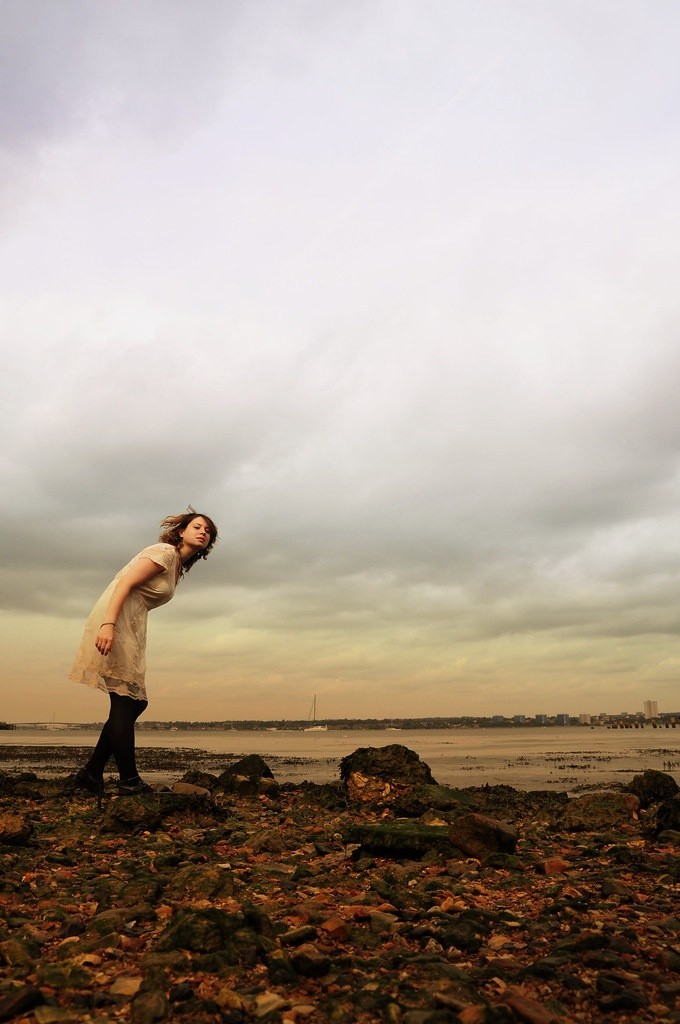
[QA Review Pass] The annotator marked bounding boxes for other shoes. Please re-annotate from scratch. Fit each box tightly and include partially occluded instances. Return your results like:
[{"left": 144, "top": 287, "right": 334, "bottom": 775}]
[
  {"left": 118, "top": 776, "right": 154, "bottom": 793},
  {"left": 72, "top": 767, "right": 105, "bottom": 793}
]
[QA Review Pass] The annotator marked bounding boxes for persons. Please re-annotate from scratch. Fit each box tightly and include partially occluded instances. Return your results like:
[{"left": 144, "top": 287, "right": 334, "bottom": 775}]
[{"left": 63, "top": 503, "right": 219, "bottom": 795}]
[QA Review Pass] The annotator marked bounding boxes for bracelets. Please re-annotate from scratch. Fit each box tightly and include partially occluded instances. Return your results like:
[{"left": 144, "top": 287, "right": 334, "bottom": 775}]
[{"left": 99, "top": 621, "right": 117, "bottom": 628}]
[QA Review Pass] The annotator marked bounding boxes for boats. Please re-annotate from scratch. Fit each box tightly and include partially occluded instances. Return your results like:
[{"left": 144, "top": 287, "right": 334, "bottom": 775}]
[{"left": 304, "top": 725, "right": 328, "bottom": 732}]
